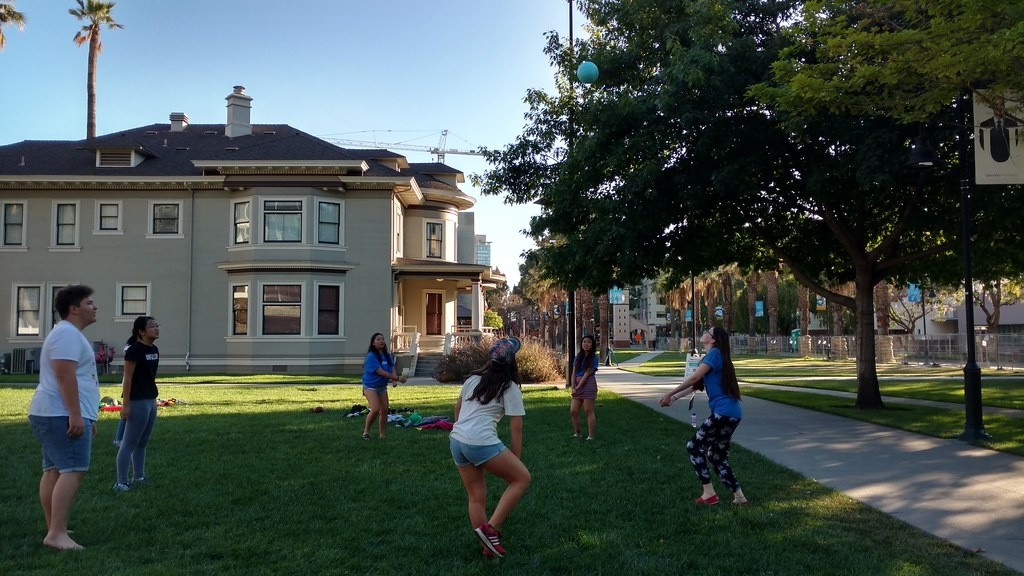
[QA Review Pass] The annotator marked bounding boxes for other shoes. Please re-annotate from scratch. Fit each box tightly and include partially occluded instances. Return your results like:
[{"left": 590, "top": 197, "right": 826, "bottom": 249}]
[
  {"left": 114, "top": 482, "right": 133, "bottom": 492},
  {"left": 572, "top": 434, "right": 582, "bottom": 438},
  {"left": 362, "top": 434, "right": 370, "bottom": 441}
]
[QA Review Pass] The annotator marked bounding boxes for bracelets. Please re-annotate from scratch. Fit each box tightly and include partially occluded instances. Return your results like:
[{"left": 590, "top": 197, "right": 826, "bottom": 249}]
[
  {"left": 673, "top": 395, "right": 677, "bottom": 401},
  {"left": 397, "top": 376, "right": 399, "bottom": 381}
]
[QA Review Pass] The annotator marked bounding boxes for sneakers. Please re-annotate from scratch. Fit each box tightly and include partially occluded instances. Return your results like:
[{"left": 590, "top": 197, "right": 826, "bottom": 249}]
[
  {"left": 696, "top": 495, "right": 720, "bottom": 506},
  {"left": 474, "top": 522, "right": 504, "bottom": 558},
  {"left": 731, "top": 499, "right": 748, "bottom": 505}
]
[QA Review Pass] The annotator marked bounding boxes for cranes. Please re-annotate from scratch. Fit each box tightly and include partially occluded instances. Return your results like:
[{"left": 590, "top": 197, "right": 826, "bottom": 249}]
[{"left": 322, "top": 129, "right": 501, "bottom": 163}]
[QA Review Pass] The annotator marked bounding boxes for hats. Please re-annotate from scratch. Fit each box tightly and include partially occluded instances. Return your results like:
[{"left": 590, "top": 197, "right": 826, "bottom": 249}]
[{"left": 489, "top": 338, "right": 521, "bottom": 363}]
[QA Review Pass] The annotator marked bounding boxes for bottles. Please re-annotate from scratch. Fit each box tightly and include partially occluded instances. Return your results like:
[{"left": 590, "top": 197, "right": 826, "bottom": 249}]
[{"left": 691, "top": 412, "right": 697, "bottom": 427}]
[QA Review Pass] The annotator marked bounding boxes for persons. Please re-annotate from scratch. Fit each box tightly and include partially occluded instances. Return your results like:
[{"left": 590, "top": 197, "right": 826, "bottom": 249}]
[
  {"left": 114, "top": 316, "right": 160, "bottom": 491},
  {"left": 28, "top": 284, "right": 101, "bottom": 551},
  {"left": 659, "top": 325, "right": 747, "bottom": 505},
  {"left": 449, "top": 337, "right": 531, "bottom": 557},
  {"left": 570, "top": 335, "right": 599, "bottom": 440},
  {"left": 362, "top": 332, "right": 407, "bottom": 440}
]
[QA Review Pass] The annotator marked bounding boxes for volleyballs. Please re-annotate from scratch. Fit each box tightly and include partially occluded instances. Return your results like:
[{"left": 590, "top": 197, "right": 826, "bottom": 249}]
[{"left": 577, "top": 61, "right": 599, "bottom": 83}]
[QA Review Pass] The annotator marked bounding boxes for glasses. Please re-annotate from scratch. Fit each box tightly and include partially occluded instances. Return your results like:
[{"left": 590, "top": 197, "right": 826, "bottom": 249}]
[
  {"left": 703, "top": 330, "right": 715, "bottom": 337},
  {"left": 144, "top": 324, "right": 160, "bottom": 329}
]
